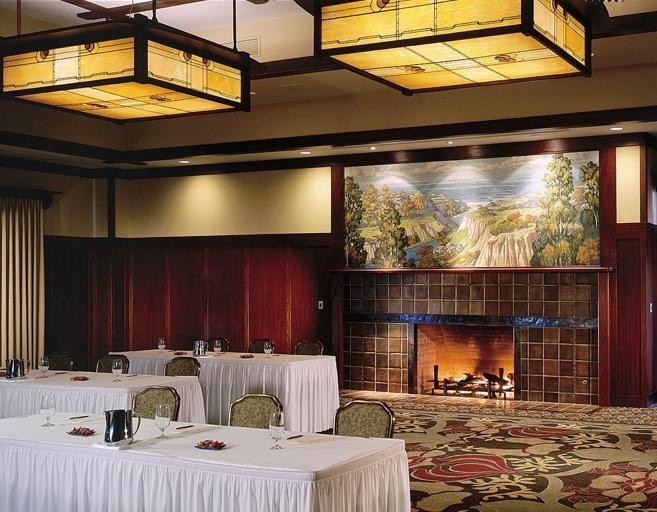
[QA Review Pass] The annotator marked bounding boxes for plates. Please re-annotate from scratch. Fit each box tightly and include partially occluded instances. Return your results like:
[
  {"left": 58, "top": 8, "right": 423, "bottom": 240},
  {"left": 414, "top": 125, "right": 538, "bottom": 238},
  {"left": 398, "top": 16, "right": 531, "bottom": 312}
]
[
  {"left": 193, "top": 440, "right": 227, "bottom": 454},
  {"left": 65, "top": 429, "right": 100, "bottom": 440}
]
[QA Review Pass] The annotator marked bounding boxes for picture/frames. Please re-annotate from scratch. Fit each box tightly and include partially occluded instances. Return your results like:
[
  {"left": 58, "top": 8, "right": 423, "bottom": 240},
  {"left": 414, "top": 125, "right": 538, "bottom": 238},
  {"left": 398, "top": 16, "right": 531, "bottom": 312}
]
[{"left": 331, "top": 136, "right": 615, "bottom": 273}]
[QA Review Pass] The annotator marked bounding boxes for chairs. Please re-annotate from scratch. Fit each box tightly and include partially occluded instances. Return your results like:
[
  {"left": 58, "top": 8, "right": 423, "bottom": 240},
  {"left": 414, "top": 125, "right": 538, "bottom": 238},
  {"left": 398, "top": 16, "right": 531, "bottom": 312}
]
[
  {"left": 333, "top": 400, "right": 396, "bottom": 438},
  {"left": 293, "top": 338, "right": 324, "bottom": 355},
  {"left": 204, "top": 337, "right": 230, "bottom": 352},
  {"left": 164, "top": 356, "right": 201, "bottom": 377},
  {"left": 43, "top": 351, "right": 73, "bottom": 371},
  {"left": 248, "top": 337, "right": 275, "bottom": 354},
  {"left": 96, "top": 354, "right": 129, "bottom": 374},
  {"left": 132, "top": 385, "right": 180, "bottom": 422},
  {"left": 228, "top": 393, "right": 284, "bottom": 429}
]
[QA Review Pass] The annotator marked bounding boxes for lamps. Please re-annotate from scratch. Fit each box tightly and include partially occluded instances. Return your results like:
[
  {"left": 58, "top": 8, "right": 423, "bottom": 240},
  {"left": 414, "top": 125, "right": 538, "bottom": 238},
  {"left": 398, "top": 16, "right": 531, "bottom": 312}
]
[
  {"left": 0, "top": 0, "right": 251, "bottom": 125},
  {"left": 314, "top": 0, "right": 592, "bottom": 96}
]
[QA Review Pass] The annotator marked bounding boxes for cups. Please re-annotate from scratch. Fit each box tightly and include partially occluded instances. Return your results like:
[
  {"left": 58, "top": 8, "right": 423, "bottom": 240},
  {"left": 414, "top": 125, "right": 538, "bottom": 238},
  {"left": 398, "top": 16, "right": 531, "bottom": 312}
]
[
  {"left": 192, "top": 340, "right": 209, "bottom": 357},
  {"left": 5, "top": 359, "right": 30, "bottom": 380},
  {"left": 104, "top": 409, "right": 141, "bottom": 446}
]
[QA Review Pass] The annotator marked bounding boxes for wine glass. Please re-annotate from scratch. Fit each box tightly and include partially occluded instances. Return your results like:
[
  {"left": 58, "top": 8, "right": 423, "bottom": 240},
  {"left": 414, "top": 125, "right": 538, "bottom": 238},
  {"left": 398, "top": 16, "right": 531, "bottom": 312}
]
[
  {"left": 38, "top": 360, "right": 50, "bottom": 378},
  {"left": 212, "top": 340, "right": 223, "bottom": 358},
  {"left": 111, "top": 358, "right": 122, "bottom": 383},
  {"left": 267, "top": 410, "right": 285, "bottom": 451},
  {"left": 39, "top": 394, "right": 57, "bottom": 427},
  {"left": 157, "top": 337, "right": 165, "bottom": 354},
  {"left": 263, "top": 340, "right": 273, "bottom": 360},
  {"left": 153, "top": 403, "right": 172, "bottom": 441}
]
